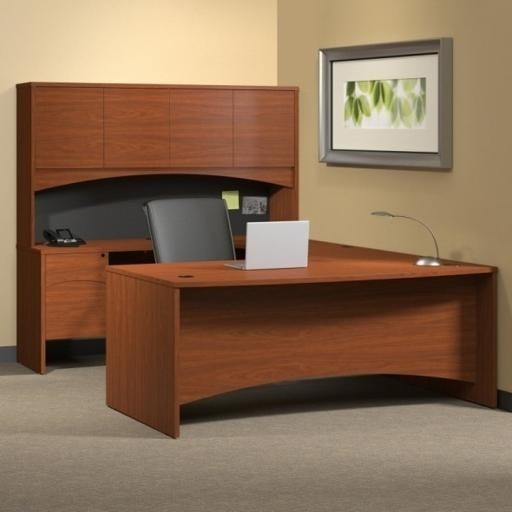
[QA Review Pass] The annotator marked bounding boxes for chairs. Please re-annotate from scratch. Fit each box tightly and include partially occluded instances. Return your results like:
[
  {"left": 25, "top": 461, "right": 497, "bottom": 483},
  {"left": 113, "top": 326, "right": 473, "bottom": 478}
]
[{"left": 141, "top": 195, "right": 234, "bottom": 264}]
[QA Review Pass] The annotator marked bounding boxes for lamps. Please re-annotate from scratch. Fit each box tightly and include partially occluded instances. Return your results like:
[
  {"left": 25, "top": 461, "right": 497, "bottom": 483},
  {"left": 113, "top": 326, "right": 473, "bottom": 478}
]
[{"left": 369, "top": 210, "right": 444, "bottom": 267}]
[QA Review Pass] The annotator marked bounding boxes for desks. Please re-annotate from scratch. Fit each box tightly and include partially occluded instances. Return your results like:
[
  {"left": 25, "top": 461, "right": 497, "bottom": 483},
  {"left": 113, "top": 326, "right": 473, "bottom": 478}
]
[{"left": 105, "top": 238, "right": 498, "bottom": 438}]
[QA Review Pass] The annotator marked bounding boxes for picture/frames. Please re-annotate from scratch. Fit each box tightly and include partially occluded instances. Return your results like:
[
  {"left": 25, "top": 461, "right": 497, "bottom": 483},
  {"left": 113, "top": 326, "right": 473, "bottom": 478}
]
[{"left": 318, "top": 36, "right": 453, "bottom": 170}]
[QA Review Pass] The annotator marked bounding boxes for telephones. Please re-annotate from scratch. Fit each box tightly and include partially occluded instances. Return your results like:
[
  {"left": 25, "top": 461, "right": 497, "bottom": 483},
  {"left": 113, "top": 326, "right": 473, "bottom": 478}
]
[{"left": 43, "top": 228, "right": 86, "bottom": 246}]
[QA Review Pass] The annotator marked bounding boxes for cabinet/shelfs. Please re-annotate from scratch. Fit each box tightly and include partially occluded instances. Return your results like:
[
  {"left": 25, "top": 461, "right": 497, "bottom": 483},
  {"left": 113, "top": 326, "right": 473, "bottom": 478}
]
[{"left": 15, "top": 81, "right": 300, "bottom": 375}]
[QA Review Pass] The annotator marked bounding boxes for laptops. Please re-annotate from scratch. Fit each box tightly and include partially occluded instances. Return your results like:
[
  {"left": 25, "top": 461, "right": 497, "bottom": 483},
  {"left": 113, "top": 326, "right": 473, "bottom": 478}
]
[{"left": 224, "top": 220, "right": 309, "bottom": 270}]
